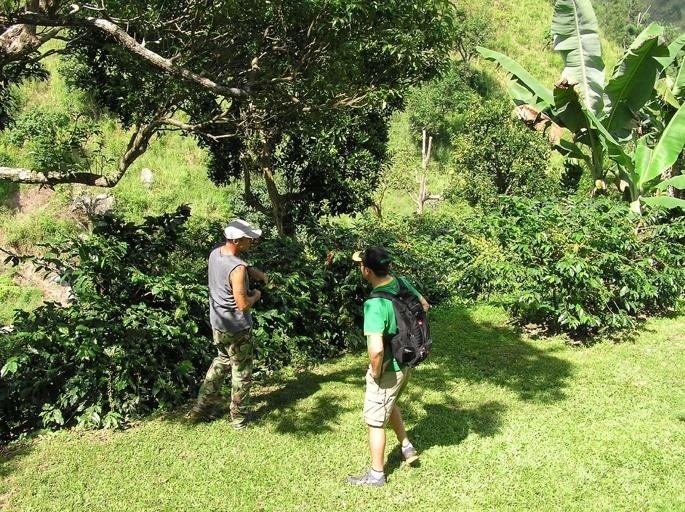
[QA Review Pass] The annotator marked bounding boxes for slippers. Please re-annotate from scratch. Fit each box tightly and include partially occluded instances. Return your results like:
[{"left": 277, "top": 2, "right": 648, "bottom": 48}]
[{"left": 369, "top": 276, "right": 433, "bottom": 369}]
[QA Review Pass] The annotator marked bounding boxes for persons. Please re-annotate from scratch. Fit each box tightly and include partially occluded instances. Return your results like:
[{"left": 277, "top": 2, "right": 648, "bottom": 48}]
[
  {"left": 347, "top": 244, "right": 431, "bottom": 487},
  {"left": 185, "top": 219, "right": 269, "bottom": 430}
]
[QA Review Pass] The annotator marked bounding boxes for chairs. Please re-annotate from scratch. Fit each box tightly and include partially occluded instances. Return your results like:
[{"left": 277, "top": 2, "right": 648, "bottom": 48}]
[
  {"left": 400, "top": 445, "right": 419, "bottom": 464},
  {"left": 232, "top": 416, "right": 248, "bottom": 431},
  {"left": 347, "top": 467, "right": 387, "bottom": 487},
  {"left": 190, "top": 408, "right": 224, "bottom": 419}
]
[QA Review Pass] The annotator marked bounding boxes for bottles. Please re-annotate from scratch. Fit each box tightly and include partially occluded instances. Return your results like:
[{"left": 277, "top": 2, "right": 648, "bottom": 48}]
[
  {"left": 223, "top": 219, "right": 262, "bottom": 239},
  {"left": 352, "top": 247, "right": 392, "bottom": 267}
]
